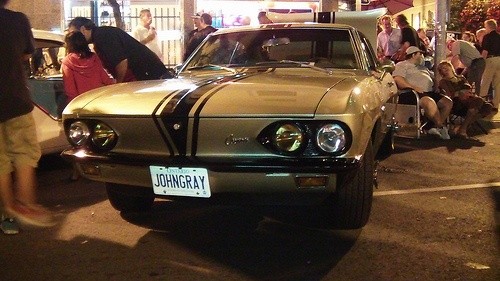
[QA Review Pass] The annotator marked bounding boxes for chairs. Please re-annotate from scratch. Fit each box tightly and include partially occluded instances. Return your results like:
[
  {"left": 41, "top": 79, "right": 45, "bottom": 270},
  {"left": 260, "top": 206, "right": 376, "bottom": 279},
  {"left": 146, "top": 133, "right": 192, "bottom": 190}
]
[{"left": 383, "top": 89, "right": 427, "bottom": 140}]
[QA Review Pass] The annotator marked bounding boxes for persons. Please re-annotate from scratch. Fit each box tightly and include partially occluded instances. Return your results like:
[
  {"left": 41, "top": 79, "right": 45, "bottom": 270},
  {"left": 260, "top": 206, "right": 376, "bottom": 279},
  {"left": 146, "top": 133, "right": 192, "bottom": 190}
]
[
  {"left": 68, "top": 15, "right": 176, "bottom": 81},
  {"left": 445, "top": 38, "right": 486, "bottom": 97},
  {"left": 415, "top": 27, "right": 477, "bottom": 77},
  {"left": 183, "top": 8, "right": 203, "bottom": 59},
  {"left": 436, "top": 59, "right": 495, "bottom": 141},
  {"left": 377, "top": 15, "right": 404, "bottom": 63},
  {"left": 0, "top": 0, "right": 57, "bottom": 234},
  {"left": 134, "top": 7, "right": 163, "bottom": 67},
  {"left": 262, "top": 36, "right": 294, "bottom": 61},
  {"left": 475, "top": 27, "right": 487, "bottom": 54},
  {"left": 390, "top": 45, "right": 453, "bottom": 141},
  {"left": 392, "top": 12, "right": 421, "bottom": 63},
  {"left": 221, "top": 11, "right": 273, "bottom": 27},
  {"left": 479, "top": 17, "right": 500, "bottom": 110},
  {"left": 61, "top": 30, "right": 117, "bottom": 103},
  {"left": 184, "top": 12, "right": 218, "bottom": 64}
]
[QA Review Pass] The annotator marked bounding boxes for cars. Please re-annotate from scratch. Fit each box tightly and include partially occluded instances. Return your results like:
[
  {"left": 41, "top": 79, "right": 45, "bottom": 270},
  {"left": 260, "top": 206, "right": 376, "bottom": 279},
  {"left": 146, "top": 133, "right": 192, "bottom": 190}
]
[
  {"left": 22, "top": 29, "right": 116, "bottom": 155},
  {"left": 61, "top": 21, "right": 398, "bottom": 230}
]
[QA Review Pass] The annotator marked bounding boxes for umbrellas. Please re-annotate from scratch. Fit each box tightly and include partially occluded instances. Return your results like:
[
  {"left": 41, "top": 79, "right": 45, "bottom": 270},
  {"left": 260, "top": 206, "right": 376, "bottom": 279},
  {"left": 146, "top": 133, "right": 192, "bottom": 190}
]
[{"left": 361, "top": 0, "right": 414, "bottom": 17}]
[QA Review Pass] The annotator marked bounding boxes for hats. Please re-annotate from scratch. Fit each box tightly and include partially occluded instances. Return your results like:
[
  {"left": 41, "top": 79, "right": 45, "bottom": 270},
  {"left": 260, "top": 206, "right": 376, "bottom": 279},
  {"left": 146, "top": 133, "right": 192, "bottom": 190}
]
[
  {"left": 405, "top": 46, "right": 421, "bottom": 55},
  {"left": 446, "top": 39, "right": 455, "bottom": 46},
  {"left": 191, "top": 10, "right": 206, "bottom": 18}
]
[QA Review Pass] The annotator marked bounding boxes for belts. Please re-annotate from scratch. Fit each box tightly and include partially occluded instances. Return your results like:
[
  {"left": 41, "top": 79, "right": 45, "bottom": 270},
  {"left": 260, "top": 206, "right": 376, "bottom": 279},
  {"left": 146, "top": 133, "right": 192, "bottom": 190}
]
[
  {"left": 471, "top": 58, "right": 483, "bottom": 63},
  {"left": 486, "top": 55, "right": 500, "bottom": 58}
]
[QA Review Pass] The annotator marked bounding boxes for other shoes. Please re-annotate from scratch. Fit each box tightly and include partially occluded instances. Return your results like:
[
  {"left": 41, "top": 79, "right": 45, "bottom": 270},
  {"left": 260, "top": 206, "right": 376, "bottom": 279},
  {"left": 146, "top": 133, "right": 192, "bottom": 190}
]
[
  {"left": 1, "top": 218, "right": 19, "bottom": 234},
  {"left": 428, "top": 127, "right": 450, "bottom": 140}
]
